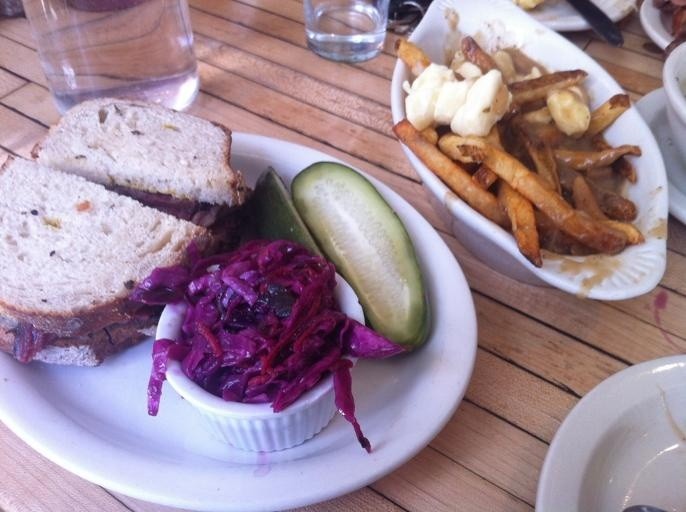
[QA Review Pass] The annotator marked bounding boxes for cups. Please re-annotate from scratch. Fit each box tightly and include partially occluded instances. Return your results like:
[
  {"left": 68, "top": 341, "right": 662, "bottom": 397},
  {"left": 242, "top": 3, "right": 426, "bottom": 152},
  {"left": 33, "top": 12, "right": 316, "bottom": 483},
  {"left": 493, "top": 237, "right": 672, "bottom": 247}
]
[
  {"left": 146, "top": 263, "right": 370, "bottom": 457},
  {"left": 19, "top": 1, "right": 202, "bottom": 123},
  {"left": 304, "top": 0, "right": 389, "bottom": 63}
]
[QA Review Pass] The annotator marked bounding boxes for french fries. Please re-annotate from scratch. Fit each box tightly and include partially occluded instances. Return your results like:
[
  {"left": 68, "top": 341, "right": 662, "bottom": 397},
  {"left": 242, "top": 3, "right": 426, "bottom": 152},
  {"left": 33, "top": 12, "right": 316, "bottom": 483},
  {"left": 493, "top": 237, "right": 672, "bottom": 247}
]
[{"left": 391, "top": 38, "right": 644, "bottom": 269}]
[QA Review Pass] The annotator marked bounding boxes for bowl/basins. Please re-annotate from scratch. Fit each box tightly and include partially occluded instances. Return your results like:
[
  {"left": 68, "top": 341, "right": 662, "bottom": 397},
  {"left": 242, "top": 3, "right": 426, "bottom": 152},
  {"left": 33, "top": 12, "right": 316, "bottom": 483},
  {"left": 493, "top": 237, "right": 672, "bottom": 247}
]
[
  {"left": 660, "top": 36, "right": 686, "bottom": 156},
  {"left": 392, "top": 2, "right": 668, "bottom": 299}
]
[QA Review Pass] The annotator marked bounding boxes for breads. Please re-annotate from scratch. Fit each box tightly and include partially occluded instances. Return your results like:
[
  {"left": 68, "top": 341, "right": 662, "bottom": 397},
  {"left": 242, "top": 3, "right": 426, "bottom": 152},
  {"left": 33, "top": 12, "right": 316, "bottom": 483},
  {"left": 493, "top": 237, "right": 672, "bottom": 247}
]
[{"left": 0, "top": 94, "right": 250, "bottom": 368}]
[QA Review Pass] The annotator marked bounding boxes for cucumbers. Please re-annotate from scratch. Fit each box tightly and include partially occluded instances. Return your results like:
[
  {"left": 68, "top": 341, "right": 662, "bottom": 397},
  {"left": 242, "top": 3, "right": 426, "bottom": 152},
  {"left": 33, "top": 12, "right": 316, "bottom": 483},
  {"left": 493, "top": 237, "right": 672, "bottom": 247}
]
[{"left": 252, "top": 161, "right": 431, "bottom": 351}]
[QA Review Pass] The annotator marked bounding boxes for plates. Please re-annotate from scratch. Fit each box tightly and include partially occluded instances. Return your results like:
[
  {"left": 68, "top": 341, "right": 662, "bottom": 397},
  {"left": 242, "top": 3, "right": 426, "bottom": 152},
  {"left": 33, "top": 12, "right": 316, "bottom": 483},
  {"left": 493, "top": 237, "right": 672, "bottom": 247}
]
[
  {"left": 638, "top": 0, "right": 685, "bottom": 50},
  {"left": 0, "top": 133, "right": 480, "bottom": 509},
  {"left": 521, "top": 0, "right": 633, "bottom": 31},
  {"left": 636, "top": 84, "right": 686, "bottom": 226},
  {"left": 519, "top": 351, "right": 685, "bottom": 511}
]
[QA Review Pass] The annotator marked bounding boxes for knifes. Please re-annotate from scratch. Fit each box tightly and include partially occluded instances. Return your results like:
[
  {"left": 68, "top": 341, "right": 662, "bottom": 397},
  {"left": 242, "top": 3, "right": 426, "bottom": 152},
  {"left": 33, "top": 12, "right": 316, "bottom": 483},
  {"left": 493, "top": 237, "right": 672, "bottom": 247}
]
[{"left": 569, "top": 1, "right": 626, "bottom": 48}]
